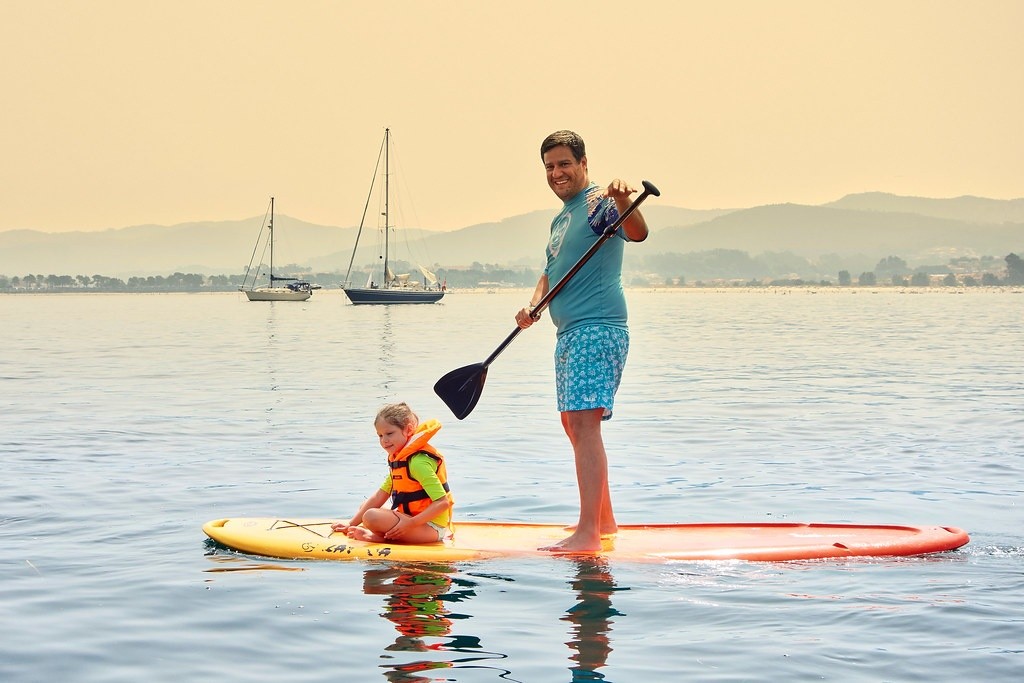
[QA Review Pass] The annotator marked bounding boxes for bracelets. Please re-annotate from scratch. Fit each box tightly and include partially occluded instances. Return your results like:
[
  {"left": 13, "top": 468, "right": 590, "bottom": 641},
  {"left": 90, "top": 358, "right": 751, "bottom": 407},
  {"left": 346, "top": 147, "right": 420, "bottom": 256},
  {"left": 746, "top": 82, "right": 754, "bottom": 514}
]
[{"left": 528, "top": 305, "right": 541, "bottom": 317}]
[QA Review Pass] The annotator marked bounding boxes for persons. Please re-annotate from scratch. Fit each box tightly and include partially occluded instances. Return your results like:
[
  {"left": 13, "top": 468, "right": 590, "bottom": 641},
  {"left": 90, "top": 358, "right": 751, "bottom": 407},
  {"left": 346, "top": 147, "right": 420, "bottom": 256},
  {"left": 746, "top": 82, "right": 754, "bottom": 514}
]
[
  {"left": 515, "top": 130, "right": 649, "bottom": 551},
  {"left": 438, "top": 283, "right": 441, "bottom": 291},
  {"left": 371, "top": 281, "right": 374, "bottom": 288},
  {"left": 331, "top": 402, "right": 452, "bottom": 543}
]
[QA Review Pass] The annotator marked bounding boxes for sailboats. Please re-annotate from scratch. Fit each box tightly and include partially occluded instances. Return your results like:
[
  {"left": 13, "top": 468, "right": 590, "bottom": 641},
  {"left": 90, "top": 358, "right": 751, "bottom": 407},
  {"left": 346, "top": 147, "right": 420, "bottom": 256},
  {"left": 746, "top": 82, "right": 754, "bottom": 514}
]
[
  {"left": 339, "top": 128, "right": 447, "bottom": 305},
  {"left": 238, "top": 196, "right": 313, "bottom": 302}
]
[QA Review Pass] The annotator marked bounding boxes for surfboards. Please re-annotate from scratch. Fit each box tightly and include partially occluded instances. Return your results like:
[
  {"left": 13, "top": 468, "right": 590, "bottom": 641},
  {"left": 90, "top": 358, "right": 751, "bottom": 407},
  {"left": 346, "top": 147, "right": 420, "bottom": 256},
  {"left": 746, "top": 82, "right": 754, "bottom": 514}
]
[{"left": 200, "top": 517, "right": 971, "bottom": 561}]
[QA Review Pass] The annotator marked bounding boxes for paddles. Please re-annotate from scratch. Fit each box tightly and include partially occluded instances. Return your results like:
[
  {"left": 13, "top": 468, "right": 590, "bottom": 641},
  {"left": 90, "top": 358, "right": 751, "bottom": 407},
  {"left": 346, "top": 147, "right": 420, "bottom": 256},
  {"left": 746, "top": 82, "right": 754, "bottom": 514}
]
[{"left": 434, "top": 178, "right": 659, "bottom": 418}]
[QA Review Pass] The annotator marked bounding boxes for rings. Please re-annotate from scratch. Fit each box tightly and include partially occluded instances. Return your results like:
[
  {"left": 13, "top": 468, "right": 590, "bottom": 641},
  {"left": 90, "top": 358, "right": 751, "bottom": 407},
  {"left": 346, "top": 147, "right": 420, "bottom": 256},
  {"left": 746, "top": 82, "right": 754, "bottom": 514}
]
[{"left": 518, "top": 320, "right": 521, "bottom": 323}]
